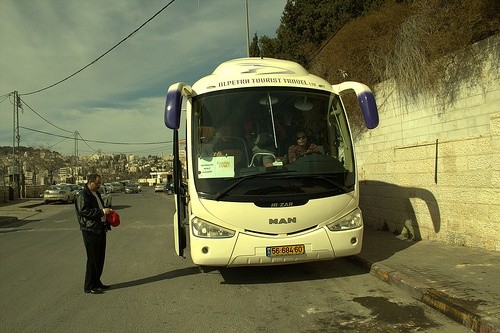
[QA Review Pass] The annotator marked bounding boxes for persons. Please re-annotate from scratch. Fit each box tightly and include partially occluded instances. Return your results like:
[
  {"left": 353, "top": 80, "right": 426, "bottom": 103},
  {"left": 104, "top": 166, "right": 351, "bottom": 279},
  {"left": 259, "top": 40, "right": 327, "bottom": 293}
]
[
  {"left": 239, "top": 118, "right": 274, "bottom": 167},
  {"left": 288, "top": 132, "right": 322, "bottom": 164},
  {"left": 75, "top": 174, "right": 112, "bottom": 294}
]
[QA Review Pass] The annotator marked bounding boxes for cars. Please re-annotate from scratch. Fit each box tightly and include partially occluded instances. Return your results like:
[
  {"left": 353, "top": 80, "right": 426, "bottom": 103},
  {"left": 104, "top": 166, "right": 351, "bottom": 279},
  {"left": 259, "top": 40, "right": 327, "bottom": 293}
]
[
  {"left": 124, "top": 184, "right": 138, "bottom": 194},
  {"left": 44, "top": 185, "right": 75, "bottom": 204},
  {"left": 165, "top": 183, "right": 188, "bottom": 195},
  {"left": 58, "top": 180, "right": 132, "bottom": 194},
  {"left": 135, "top": 183, "right": 142, "bottom": 192},
  {"left": 76, "top": 185, "right": 112, "bottom": 208},
  {"left": 155, "top": 184, "right": 164, "bottom": 193}
]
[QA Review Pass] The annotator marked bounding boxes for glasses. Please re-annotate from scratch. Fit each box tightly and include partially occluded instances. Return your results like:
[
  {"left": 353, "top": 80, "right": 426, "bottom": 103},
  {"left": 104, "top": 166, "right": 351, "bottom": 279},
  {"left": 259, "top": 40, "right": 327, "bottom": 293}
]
[
  {"left": 297, "top": 136, "right": 306, "bottom": 140},
  {"left": 93, "top": 181, "right": 101, "bottom": 186}
]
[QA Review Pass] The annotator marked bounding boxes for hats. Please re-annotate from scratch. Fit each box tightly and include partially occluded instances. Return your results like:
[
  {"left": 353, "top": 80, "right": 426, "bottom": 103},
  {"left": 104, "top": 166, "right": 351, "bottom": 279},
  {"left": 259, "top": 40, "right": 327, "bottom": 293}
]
[{"left": 106, "top": 210, "right": 121, "bottom": 227}]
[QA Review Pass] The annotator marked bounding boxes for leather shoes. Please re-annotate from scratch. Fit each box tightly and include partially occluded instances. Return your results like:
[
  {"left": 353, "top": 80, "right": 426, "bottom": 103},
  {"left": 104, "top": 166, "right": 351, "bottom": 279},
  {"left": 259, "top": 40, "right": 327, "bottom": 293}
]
[{"left": 84, "top": 283, "right": 110, "bottom": 295}]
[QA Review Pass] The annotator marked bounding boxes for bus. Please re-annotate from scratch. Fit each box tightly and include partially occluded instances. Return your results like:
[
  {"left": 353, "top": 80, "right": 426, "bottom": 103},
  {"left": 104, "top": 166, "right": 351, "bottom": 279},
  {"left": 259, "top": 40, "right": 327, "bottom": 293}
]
[{"left": 164, "top": 57, "right": 379, "bottom": 266}]
